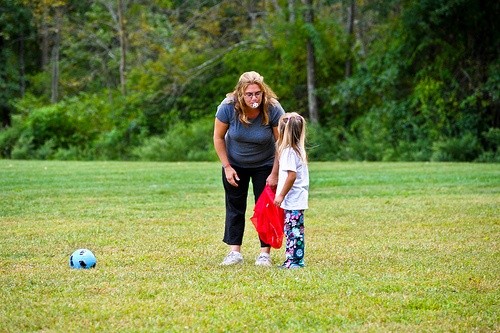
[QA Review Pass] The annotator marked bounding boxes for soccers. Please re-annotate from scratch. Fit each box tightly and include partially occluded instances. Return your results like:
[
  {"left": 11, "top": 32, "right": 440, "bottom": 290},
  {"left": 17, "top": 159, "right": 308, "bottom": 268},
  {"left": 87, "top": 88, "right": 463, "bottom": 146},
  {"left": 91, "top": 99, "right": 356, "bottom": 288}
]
[{"left": 69, "top": 249, "right": 96, "bottom": 269}]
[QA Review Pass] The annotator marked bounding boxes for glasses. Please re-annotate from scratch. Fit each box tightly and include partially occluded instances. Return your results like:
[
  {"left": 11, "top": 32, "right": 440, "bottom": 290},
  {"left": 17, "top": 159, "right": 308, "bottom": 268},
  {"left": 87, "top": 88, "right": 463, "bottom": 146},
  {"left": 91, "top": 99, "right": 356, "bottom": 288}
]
[{"left": 245, "top": 92, "right": 262, "bottom": 98}]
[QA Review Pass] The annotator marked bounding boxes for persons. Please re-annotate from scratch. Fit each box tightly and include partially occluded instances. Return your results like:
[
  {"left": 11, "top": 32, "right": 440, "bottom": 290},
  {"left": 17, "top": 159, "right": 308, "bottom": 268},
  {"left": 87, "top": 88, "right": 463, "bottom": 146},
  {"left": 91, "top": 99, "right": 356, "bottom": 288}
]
[
  {"left": 274, "top": 112, "right": 310, "bottom": 272},
  {"left": 213, "top": 70, "right": 286, "bottom": 268}
]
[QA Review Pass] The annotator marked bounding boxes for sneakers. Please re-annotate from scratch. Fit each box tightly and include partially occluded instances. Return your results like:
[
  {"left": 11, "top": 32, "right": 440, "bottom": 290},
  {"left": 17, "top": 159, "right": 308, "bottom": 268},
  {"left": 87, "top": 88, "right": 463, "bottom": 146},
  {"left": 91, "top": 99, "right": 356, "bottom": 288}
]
[
  {"left": 219, "top": 251, "right": 243, "bottom": 266},
  {"left": 254, "top": 252, "right": 272, "bottom": 268}
]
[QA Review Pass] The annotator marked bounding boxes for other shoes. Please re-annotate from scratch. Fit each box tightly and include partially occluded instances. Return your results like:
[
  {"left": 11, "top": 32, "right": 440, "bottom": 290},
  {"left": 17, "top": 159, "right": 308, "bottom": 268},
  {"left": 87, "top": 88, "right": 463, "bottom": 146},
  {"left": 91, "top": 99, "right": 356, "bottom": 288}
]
[{"left": 278, "top": 266, "right": 286, "bottom": 269}]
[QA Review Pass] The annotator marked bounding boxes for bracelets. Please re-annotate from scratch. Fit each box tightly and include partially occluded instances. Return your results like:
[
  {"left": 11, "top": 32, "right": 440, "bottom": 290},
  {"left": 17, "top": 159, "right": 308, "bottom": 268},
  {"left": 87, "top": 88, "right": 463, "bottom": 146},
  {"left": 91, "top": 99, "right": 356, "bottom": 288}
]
[{"left": 222, "top": 163, "right": 230, "bottom": 169}]
